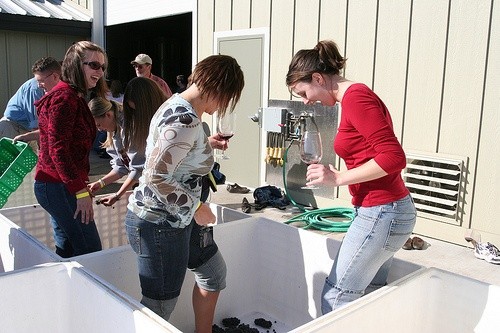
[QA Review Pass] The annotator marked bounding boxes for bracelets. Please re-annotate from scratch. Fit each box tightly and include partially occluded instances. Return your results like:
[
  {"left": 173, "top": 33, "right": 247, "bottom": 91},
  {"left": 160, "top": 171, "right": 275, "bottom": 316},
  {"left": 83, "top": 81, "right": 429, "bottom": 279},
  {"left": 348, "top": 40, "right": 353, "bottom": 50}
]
[
  {"left": 98, "top": 179, "right": 105, "bottom": 188},
  {"left": 75, "top": 192, "right": 90, "bottom": 200}
]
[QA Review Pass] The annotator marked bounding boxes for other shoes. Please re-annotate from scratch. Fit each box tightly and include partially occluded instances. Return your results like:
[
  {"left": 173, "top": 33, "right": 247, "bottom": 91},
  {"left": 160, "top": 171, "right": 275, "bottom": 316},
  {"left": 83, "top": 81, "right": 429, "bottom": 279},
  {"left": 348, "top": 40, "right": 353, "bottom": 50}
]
[
  {"left": 402, "top": 237, "right": 424, "bottom": 250},
  {"left": 242, "top": 198, "right": 251, "bottom": 213},
  {"left": 254, "top": 202, "right": 267, "bottom": 209},
  {"left": 227, "top": 183, "right": 249, "bottom": 193}
]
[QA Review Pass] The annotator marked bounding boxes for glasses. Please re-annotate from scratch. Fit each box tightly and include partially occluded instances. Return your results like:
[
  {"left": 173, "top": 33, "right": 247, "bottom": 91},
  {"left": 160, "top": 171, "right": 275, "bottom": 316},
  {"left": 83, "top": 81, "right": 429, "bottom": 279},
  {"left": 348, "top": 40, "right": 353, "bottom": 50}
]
[
  {"left": 133, "top": 64, "right": 146, "bottom": 69},
  {"left": 83, "top": 61, "right": 106, "bottom": 72},
  {"left": 37, "top": 73, "right": 53, "bottom": 84}
]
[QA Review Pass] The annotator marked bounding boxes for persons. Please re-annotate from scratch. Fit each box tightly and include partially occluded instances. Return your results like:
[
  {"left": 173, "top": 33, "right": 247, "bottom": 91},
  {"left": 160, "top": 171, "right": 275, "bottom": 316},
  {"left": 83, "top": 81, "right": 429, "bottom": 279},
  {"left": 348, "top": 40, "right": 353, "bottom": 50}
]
[
  {"left": 286, "top": 40, "right": 418, "bottom": 316},
  {"left": 132, "top": 53, "right": 173, "bottom": 102},
  {"left": 117, "top": 77, "right": 212, "bottom": 204},
  {"left": 124, "top": 55, "right": 245, "bottom": 333},
  {"left": 176, "top": 74, "right": 188, "bottom": 94},
  {"left": 84, "top": 95, "right": 148, "bottom": 206},
  {"left": 33, "top": 41, "right": 109, "bottom": 257},
  {"left": 0, "top": 54, "right": 63, "bottom": 148},
  {"left": 92, "top": 79, "right": 126, "bottom": 154}
]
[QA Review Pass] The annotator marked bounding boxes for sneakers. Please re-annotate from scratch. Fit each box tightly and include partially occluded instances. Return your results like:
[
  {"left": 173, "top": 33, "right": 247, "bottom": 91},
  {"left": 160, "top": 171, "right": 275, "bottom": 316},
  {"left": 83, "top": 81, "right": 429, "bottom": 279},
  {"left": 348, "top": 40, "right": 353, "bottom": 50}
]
[
  {"left": 474, "top": 242, "right": 500, "bottom": 265},
  {"left": 99, "top": 151, "right": 110, "bottom": 157}
]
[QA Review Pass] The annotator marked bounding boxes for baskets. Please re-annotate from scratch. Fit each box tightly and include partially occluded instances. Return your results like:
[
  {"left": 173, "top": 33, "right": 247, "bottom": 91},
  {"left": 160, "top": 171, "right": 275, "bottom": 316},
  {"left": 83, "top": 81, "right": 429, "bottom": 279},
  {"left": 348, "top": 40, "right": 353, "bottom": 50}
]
[{"left": 0, "top": 136, "right": 38, "bottom": 209}]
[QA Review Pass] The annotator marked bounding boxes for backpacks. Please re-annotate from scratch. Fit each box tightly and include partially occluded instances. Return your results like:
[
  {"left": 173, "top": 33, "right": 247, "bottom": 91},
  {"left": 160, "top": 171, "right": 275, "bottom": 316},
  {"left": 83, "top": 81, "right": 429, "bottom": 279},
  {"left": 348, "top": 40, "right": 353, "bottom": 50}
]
[{"left": 253, "top": 185, "right": 290, "bottom": 210}]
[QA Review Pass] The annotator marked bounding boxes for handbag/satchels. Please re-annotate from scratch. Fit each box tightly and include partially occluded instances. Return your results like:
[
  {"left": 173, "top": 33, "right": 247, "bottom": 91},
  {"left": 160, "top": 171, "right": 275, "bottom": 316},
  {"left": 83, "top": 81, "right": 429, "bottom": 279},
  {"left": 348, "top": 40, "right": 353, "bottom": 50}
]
[{"left": 213, "top": 162, "right": 226, "bottom": 185}]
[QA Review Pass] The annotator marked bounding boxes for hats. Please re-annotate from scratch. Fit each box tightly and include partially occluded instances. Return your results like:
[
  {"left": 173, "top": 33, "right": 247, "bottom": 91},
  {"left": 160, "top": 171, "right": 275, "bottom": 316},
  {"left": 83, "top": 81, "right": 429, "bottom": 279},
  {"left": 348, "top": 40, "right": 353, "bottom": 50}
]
[{"left": 131, "top": 54, "right": 152, "bottom": 64}]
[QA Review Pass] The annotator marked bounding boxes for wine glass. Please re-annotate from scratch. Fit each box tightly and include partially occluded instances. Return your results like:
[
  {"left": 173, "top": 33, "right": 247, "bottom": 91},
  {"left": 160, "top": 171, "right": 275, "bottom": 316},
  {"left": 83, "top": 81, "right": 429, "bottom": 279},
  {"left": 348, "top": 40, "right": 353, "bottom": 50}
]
[
  {"left": 299, "top": 130, "right": 323, "bottom": 190},
  {"left": 214, "top": 112, "right": 237, "bottom": 159}
]
